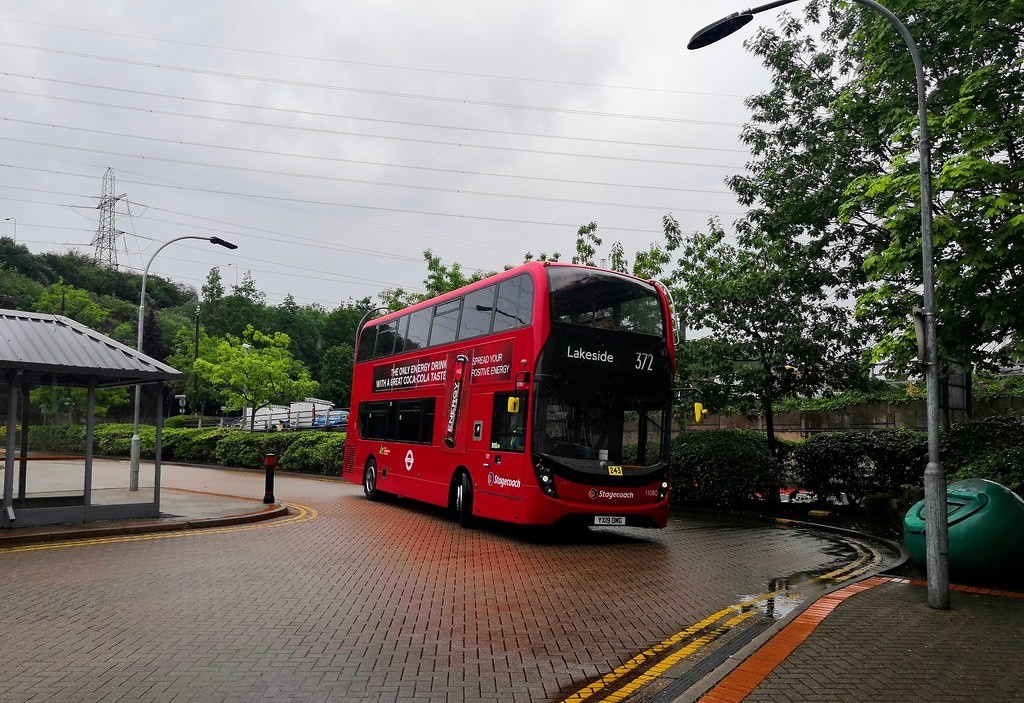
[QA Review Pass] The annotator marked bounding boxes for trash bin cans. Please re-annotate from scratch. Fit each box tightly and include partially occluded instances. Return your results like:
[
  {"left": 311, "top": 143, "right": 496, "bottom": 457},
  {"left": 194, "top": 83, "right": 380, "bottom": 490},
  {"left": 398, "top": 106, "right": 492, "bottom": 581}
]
[{"left": 902, "top": 481, "right": 1024, "bottom": 577}]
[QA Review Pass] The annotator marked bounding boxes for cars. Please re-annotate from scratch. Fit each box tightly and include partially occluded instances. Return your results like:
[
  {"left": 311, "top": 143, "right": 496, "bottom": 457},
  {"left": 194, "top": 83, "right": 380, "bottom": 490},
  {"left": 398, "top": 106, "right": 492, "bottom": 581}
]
[{"left": 317, "top": 411, "right": 349, "bottom": 427}]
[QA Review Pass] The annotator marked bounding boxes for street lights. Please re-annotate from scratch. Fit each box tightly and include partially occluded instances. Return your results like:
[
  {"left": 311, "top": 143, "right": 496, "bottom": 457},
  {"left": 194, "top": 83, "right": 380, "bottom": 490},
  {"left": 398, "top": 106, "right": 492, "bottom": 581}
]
[
  {"left": 688, "top": 0, "right": 950, "bottom": 609},
  {"left": 476, "top": 305, "right": 527, "bottom": 325},
  {"left": 5, "top": 217, "right": 16, "bottom": 243},
  {"left": 228, "top": 262, "right": 238, "bottom": 287},
  {"left": 129, "top": 236, "right": 238, "bottom": 490}
]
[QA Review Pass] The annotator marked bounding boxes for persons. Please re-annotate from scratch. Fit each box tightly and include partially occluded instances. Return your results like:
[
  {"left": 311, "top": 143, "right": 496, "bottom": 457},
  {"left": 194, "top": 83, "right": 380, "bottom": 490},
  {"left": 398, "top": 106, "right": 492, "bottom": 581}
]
[
  {"left": 599, "top": 312, "right": 616, "bottom": 330},
  {"left": 509, "top": 414, "right": 544, "bottom": 454}
]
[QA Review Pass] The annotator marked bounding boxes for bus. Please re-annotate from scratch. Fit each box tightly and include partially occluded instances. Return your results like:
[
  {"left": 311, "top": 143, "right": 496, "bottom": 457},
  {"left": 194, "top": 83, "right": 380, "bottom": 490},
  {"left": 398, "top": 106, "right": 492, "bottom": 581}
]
[{"left": 343, "top": 261, "right": 708, "bottom": 529}]
